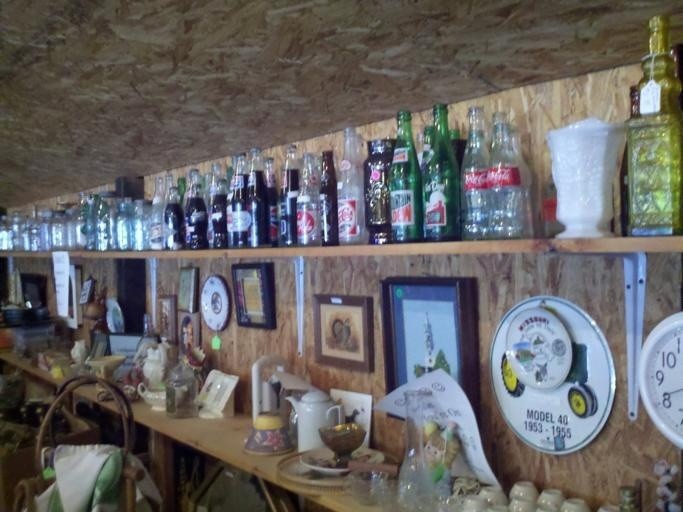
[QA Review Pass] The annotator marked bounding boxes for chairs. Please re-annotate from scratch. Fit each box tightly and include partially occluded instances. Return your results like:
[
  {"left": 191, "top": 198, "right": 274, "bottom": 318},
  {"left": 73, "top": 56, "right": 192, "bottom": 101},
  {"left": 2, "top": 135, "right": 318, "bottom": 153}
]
[{"left": 37, "top": 445, "right": 146, "bottom": 511}]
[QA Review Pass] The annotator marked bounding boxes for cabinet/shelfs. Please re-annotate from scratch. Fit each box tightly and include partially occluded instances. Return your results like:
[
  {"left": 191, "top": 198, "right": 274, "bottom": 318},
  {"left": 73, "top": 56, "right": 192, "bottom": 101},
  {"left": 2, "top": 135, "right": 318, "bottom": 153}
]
[{"left": 0, "top": 234, "right": 683, "bottom": 511}]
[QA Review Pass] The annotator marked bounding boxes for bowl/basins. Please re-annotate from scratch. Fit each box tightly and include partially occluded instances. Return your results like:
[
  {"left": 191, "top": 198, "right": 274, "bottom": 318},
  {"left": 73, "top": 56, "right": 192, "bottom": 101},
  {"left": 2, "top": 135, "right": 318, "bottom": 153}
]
[
  {"left": 238, "top": 410, "right": 294, "bottom": 455},
  {"left": 2, "top": 308, "right": 39, "bottom": 329},
  {"left": 87, "top": 353, "right": 127, "bottom": 379}
]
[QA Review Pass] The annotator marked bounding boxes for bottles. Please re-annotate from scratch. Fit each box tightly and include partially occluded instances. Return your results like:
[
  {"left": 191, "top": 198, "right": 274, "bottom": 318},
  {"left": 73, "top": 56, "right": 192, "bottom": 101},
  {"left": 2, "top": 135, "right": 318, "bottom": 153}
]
[
  {"left": 163, "top": 360, "right": 200, "bottom": 419},
  {"left": 397, "top": 390, "right": 437, "bottom": 512},
  {"left": 0, "top": 99, "right": 536, "bottom": 254},
  {"left": 137, "top": 313, "right": 160, "bottom": 355},
  {"left": 618, "top": 15, "right": 683, "bottom": 237},
  {"left": 619, "top": 484, "right": 639, "bottom": 512}
]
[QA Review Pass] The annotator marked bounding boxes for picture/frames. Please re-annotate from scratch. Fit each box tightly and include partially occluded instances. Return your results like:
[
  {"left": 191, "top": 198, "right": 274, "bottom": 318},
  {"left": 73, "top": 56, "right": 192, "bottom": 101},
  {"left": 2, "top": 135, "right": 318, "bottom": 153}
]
[
  {"left": 312, "top": 294, "right": 374, "bottom": 373},
  {"left": 232, "top": 263, "right": 276, "bottom": 329},
  {"left": 381, "top": 277, "right": 482, "bottom": 429}
]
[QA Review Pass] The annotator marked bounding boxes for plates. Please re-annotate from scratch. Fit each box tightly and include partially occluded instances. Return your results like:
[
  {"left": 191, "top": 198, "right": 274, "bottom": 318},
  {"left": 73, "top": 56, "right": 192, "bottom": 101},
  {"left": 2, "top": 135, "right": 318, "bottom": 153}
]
[
  {"left": 298, "top": 447, "right": 385, "bottom": 477},
  {"left": 200, "top": 275, "right": 231, "bottom": 331},
  {"left": 104, "top": 302, "right": 127, "bottom": 335},
  {"left": 504, "top": 304, "right": 575, "bottom": 395}
]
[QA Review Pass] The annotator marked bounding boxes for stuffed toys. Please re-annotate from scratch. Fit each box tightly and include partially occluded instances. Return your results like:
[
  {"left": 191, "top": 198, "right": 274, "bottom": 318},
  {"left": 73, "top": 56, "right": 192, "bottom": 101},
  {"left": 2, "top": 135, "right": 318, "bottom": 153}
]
[{"left": 423, "top": 421, "right": 461, "bottom": 482}]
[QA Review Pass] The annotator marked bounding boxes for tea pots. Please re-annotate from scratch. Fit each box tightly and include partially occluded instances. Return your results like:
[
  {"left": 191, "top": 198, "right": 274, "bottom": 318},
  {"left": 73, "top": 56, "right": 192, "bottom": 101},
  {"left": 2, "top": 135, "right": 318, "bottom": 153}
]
[
  {"left": 136, "top": 384, "right": 189, "bottom": 413},
  {"left": 283, "top": 388, "right": 345, "bottom": 454}
]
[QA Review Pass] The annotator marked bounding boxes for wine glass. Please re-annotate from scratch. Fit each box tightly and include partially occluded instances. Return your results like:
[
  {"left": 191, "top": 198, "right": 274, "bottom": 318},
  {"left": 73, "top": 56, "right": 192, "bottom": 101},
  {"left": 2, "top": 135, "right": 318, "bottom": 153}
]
[{"left": 318, "top": 422, "right": 368, "bottom": 468}]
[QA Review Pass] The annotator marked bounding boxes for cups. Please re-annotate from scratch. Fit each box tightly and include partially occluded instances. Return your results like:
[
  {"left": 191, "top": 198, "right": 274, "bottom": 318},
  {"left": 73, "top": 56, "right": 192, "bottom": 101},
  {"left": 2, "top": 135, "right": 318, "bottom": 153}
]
[
  {"left": 461, "top": 479, "right": 619, "bottom": 512},
  {"left": 545, "top": 115, "right": 623, "bottom": 242},
  {"left": 340, "top": 461, "right": 480, "bottom": 512}
]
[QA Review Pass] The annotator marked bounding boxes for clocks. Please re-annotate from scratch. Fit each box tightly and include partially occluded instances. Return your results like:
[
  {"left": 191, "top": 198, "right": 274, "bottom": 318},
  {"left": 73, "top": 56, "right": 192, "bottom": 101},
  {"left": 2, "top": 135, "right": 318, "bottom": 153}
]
[{"left": 636, "top": 311, "right": 683, "bottom": 451}]
